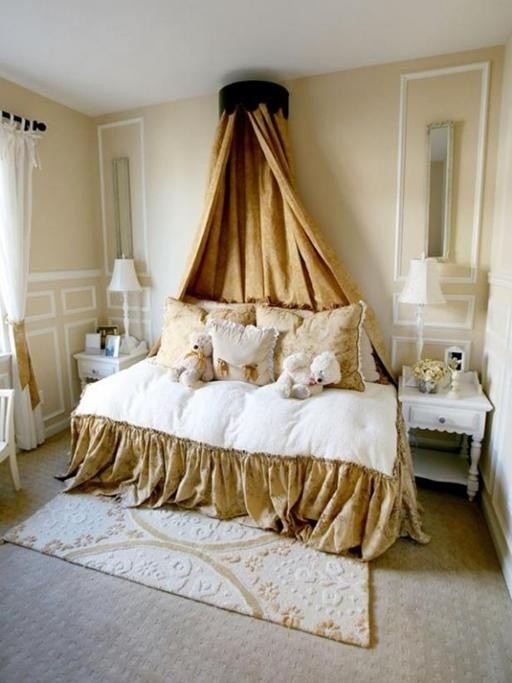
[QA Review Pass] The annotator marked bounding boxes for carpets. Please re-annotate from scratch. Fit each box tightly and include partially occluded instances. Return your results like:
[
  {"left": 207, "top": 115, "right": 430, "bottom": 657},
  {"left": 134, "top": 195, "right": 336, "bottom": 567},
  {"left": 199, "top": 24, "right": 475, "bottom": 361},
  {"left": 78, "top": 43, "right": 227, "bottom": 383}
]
[{"left": 1, "top": 487, "right": 379, "bottom": 649}]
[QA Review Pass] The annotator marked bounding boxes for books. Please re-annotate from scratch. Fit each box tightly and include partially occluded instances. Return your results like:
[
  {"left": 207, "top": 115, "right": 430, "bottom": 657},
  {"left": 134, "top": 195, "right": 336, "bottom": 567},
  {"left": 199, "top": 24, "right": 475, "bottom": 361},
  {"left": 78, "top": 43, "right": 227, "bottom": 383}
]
[
  {"left": 104, "top": 334, "right": 121, "bottom": 358},
  {"left": 83, "top": 332, "right": 102, "bottom": 355}
]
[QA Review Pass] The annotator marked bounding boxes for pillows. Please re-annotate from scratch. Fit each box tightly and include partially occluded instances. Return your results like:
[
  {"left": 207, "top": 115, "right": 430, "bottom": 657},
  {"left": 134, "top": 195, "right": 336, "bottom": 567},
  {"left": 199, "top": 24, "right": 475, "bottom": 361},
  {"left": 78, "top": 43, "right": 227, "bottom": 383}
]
[{"left": 152, "top": 293, "right": 382, "bottom": 394}]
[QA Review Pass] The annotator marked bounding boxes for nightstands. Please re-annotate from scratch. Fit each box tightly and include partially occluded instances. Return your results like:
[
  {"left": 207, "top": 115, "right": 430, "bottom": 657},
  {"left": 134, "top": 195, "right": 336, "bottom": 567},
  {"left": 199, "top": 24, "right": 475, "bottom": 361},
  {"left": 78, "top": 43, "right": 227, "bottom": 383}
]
[
  {"left": 395, "top": 366, "right": 496, "bottom": 503},
  {"left": 71, "top": 348, "right": 150, "bottom": 392}
]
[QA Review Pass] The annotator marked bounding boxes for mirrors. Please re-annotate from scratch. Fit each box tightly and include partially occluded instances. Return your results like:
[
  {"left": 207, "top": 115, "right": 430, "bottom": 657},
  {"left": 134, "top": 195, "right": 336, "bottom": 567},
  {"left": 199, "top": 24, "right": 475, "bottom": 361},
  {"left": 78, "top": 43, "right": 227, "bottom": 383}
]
[
  {"left": 111, "top": 155, "right": 137, "bottom": 259},
  {"left": 420, "top": 117, "right": 458, "bottom": 265},
  {"left": 391, "top": 55, "right": 492, "bottom": 286},
  {"left": 93, "top": 114, "right": 150, "bottom": 281}
]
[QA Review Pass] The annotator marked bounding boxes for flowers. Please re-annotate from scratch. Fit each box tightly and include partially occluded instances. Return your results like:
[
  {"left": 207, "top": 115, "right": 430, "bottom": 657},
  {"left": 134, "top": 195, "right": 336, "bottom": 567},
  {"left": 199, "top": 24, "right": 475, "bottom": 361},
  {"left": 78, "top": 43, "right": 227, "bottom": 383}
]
[{"left": 410, "top": 356, "right": 447, "bottom": 382}]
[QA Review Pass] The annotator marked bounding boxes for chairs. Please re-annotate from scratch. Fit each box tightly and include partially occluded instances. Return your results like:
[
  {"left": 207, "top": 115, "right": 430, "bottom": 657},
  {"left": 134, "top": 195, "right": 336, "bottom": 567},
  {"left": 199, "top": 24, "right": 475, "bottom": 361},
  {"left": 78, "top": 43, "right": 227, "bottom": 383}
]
[{"left": 0, "top": 388, "right": 24, "bottom": 493}]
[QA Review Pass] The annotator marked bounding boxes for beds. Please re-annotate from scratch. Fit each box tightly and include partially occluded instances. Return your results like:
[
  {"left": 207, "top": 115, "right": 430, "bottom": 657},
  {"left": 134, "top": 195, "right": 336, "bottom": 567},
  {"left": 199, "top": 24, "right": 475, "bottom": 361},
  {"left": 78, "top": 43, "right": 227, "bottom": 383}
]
[{"left": 62, "top": 288, "right": 433, "bottom": 564}]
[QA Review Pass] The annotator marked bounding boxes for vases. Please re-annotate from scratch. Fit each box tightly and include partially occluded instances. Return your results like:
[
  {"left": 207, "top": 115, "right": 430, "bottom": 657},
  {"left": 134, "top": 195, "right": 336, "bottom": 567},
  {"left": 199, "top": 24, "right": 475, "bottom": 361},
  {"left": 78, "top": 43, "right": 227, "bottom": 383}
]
[{"left": 417, "top": 376, "right": 440, "bottom": 393}]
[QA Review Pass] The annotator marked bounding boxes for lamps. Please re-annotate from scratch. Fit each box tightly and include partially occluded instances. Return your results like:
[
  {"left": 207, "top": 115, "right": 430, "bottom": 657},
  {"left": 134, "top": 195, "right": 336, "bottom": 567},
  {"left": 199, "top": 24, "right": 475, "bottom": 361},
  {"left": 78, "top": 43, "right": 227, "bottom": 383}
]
[
  {"left": 398, "top": 247, "right": 449, "bottom": 389},
  {"left": 104, "top": 251, "right": 146, "bottom": 339}
]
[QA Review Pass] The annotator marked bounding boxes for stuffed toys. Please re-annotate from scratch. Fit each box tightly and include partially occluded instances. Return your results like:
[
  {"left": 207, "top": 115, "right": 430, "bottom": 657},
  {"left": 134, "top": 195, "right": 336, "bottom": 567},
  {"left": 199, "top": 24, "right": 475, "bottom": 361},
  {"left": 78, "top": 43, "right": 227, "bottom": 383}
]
[
  {"left": 273, "top": 349, "right": 343, "bottom": 398},
  {"left": 166, "top": 331, "right": 214, "bottom": 389}
]
